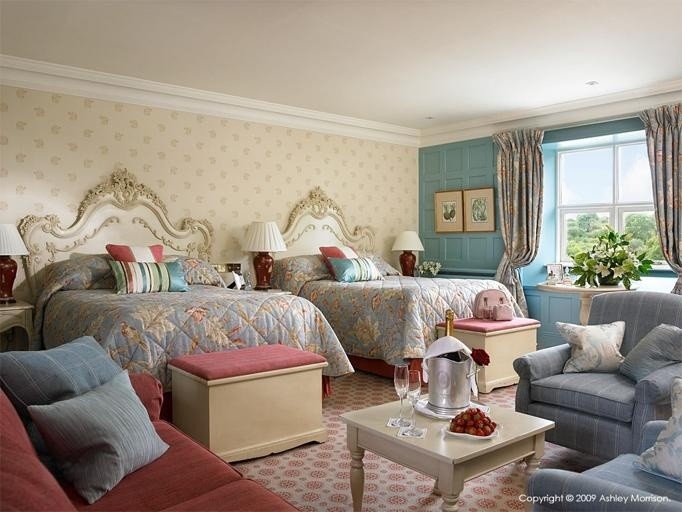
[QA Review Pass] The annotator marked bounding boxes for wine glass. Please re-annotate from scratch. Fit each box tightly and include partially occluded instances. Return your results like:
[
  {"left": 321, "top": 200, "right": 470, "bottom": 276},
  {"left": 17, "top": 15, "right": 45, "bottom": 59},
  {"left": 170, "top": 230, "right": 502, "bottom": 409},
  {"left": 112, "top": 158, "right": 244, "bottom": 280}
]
[
  {"left": 403, "top": 369, "right": 422, "bottom": 437},
  {"left": 393, "top": 362, "right": 409, "bottom": 427}
]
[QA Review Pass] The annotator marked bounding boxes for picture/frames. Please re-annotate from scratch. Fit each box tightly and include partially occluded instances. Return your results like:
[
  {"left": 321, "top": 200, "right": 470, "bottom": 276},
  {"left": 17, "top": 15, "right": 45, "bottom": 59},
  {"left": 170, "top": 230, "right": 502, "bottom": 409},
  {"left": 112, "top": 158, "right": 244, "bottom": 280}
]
[
  {"left": 547, "top": 264, "right": 563, "bottom": 283},
  {"left": 463, "top": 187, "right": 494, "bottom": 232},
  {"left": 434, "top": 189, "right": 463, "bottom": 233}
]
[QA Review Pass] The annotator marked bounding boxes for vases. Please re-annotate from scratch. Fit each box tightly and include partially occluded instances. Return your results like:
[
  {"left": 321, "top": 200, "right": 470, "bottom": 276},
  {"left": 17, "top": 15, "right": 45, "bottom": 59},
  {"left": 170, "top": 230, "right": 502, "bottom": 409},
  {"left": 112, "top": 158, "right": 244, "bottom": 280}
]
[{"left": 597, "top": 271, "right": 622, "bottom": 286}]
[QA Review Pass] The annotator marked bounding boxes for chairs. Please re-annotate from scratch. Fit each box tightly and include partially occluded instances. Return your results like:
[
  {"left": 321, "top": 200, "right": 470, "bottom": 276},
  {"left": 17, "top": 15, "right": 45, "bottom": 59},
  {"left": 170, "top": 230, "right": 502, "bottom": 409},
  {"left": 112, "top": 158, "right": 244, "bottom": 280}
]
[
  {"left": 526, "top": 421, "right": 682, "bottom": 512},
  {"left": 512, "top": 291, "right": 682, "bottom": 459}
]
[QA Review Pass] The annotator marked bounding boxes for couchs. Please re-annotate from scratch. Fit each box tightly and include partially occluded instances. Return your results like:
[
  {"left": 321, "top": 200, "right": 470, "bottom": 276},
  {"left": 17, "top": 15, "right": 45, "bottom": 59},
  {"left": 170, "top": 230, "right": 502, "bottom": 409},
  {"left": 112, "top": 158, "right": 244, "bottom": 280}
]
[{"left": 0, "top": 369, "right": 304, "bottom": 512}]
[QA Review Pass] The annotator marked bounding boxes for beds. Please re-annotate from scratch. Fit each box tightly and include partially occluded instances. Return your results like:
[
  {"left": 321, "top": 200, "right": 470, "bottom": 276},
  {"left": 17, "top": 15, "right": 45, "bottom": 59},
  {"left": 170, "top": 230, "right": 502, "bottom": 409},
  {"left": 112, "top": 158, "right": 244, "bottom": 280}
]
[
  {"left": 245, "top": 184, "right": 527, "bottom": 382},
  {"left": 16, "top": 166, "right": 355, "bottom": 419}
]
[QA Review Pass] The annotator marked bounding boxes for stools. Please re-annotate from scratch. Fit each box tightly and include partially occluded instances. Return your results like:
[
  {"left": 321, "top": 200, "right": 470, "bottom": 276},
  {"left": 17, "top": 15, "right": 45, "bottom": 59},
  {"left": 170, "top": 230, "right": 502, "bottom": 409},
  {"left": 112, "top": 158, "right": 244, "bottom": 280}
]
[
  {"left": 435, "top": 316, "right": 542, "bottom": 393},
  {"left": 167, "top": 343, "right": 328, "bottom": 464}
]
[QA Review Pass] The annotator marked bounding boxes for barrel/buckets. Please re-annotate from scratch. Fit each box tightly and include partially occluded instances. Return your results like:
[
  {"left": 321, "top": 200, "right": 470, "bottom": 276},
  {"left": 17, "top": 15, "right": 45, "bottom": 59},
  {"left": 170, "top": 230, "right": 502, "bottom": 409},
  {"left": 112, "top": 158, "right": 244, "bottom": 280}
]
[{"left": 420, "top": 351, "right": 481, "bottom": 415}]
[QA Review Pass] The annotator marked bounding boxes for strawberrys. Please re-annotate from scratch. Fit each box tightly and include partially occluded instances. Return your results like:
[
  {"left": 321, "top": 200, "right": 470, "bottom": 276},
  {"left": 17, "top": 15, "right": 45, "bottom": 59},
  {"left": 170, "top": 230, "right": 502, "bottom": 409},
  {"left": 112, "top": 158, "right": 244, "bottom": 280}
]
[{"left": 450, "top": 408, "right": 496, "bottom": 436}]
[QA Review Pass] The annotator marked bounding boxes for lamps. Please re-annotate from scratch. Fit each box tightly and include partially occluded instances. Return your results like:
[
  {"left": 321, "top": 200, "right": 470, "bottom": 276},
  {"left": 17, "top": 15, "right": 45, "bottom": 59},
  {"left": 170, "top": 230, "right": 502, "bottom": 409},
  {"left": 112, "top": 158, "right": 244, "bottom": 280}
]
[
  {"left": 392, "top": 230, "right": 424, "bottom": 277},
  {"left": 0, "top": 224, "right": 29, "bottom": 305},
  {"left": 241, "top": 223, "right": 288, "bottom": 289}
]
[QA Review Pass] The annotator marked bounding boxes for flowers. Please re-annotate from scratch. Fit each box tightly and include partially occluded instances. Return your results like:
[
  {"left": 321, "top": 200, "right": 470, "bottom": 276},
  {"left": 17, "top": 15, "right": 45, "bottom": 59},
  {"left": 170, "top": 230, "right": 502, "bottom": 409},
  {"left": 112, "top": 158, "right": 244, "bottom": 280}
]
[
  {"left": 568, "top": 224, "right": 655, "bottom": 290},
  {"left": 417, "top": 260, "right": 441, "bottom": 277}
]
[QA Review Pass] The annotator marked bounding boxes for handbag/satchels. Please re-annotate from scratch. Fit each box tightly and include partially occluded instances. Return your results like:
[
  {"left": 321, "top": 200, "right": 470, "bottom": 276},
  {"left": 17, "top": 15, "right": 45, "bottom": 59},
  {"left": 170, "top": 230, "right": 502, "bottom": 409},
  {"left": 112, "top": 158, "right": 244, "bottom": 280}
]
[{"left": 474, "top": 290, "right": 513, "bottom": 320}]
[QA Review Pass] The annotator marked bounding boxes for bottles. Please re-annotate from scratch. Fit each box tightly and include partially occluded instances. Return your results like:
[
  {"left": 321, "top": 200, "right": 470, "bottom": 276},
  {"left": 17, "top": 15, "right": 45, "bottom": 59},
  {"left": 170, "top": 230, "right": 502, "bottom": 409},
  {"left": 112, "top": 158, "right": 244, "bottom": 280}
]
[
  {"left": 563, "top": 266, "right": 572, "bottom": 286},
  {"left": 238, "top": 272, "right": 244, "bottom": 289},
  {"left": 546, "top": 270, "right": 557, "bottom": 285},
  {"left": 442, "top": 309, "right": 461, "bottom": 363},
  {"left": 245, "top": 272, "right": 253, "bottom": 291}
]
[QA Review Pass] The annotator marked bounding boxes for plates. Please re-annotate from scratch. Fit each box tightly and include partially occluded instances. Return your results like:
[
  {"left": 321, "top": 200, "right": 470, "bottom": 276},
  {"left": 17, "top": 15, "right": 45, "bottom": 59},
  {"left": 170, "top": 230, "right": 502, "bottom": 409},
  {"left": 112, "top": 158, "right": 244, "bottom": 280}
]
[{"left": 445, "top": 423, "right": 501, "bottom": 441}]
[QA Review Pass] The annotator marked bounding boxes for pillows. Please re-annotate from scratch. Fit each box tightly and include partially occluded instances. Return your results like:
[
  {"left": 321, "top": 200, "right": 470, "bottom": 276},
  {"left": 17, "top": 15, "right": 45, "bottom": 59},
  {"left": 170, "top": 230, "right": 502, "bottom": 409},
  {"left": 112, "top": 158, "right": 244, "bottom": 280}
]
[
  {"left": 273, "top": 253, "right": 403, "bottom": 296},
  {"left": 619, "top": 322, "right": 682, "bottom": 381},
  {"left": 555, "top": 321, "right": 626, "bottom": 374},
  {"left": 0, "top": 335, "right": 124, "bottom": 477},
  {"left": 327, "top": 257, "right": 385, "bottom": 281},
  {"left": 319, "top": 245, "right": 360, "bottom": 277},
  {"left": 28, "top": 367, "right": 169, "bottom": 503},
  {"left": 110, "top": 260, "right": 189, "bottom": 295},
  {"left": 632, "top": 376, "right": 682, "bottom": 484},
  {"left": 35, "top": 253, "right": 228, "bottom": 292},
  {"left": 106, "top": 245, "right": 163, "bottom": 262}
]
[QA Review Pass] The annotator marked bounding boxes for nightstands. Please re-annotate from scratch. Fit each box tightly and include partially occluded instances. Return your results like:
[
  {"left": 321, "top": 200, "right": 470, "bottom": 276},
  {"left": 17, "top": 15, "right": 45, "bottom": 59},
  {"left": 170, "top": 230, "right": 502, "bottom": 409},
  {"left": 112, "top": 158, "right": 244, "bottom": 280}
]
[
  {"left": 0, "top": 301, "right": 35, "bottom": 352},
  {"left": 249, "top": 288, "right": 292, "bottom": 296}
]
